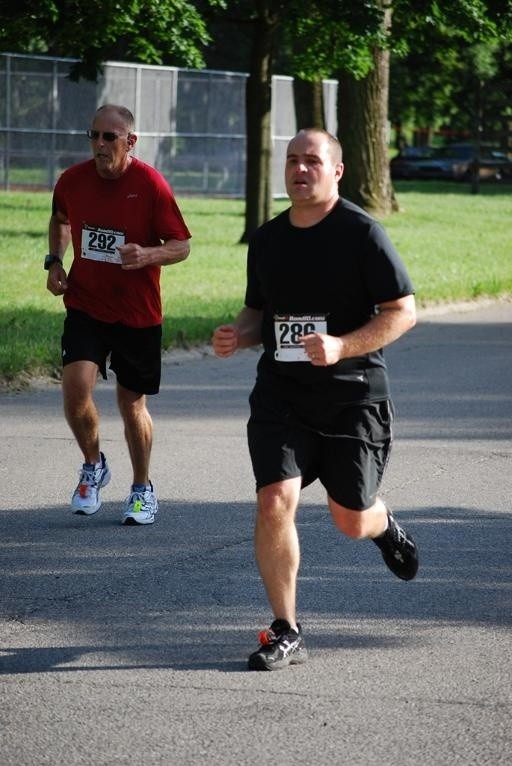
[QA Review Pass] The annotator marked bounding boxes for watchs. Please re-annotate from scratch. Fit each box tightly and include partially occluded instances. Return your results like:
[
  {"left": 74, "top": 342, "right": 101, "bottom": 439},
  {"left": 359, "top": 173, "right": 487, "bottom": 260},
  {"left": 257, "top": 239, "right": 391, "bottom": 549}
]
[{"left": 44, "top": 255, "right": 64, "bottom": 270}]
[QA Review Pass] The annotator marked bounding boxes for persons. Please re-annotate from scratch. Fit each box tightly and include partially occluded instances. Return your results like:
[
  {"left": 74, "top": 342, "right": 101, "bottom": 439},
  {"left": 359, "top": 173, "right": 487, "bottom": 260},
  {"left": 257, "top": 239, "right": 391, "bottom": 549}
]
[
  {"left": 209, "top": 124, "right": 421, "bottom": 673},
  {"left": 41, "top": 102, "right": 194, "bottom": 528}
]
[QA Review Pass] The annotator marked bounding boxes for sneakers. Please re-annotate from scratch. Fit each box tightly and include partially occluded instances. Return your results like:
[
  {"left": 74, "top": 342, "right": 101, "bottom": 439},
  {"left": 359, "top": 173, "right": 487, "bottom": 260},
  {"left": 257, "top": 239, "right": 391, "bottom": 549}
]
[
  {"left": 247, "top": 618, "right": 309, "bottom": 672},
  {"left": 120, "top": 480, "right": 158, "bottom": 526},
  {"left": 70, "top": 451, "right": 112, "bottom": 516},
  {"left": 370, "top": 508, "right": 419, "bottom": 581}
]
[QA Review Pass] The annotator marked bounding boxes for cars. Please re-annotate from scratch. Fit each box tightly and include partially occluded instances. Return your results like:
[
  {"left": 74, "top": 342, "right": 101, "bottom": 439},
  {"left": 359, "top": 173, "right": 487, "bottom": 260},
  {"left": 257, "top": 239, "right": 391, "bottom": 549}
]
[{"left": 387, "top": 142, "right": 511, "bottom": 186}]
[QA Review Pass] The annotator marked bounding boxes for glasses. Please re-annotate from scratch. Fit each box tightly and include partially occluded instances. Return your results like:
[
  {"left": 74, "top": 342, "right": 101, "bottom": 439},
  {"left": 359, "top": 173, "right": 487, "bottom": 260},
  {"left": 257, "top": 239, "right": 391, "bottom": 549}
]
[{"left": 86, "top": 127, "right": 129, "bottom": 141}]
